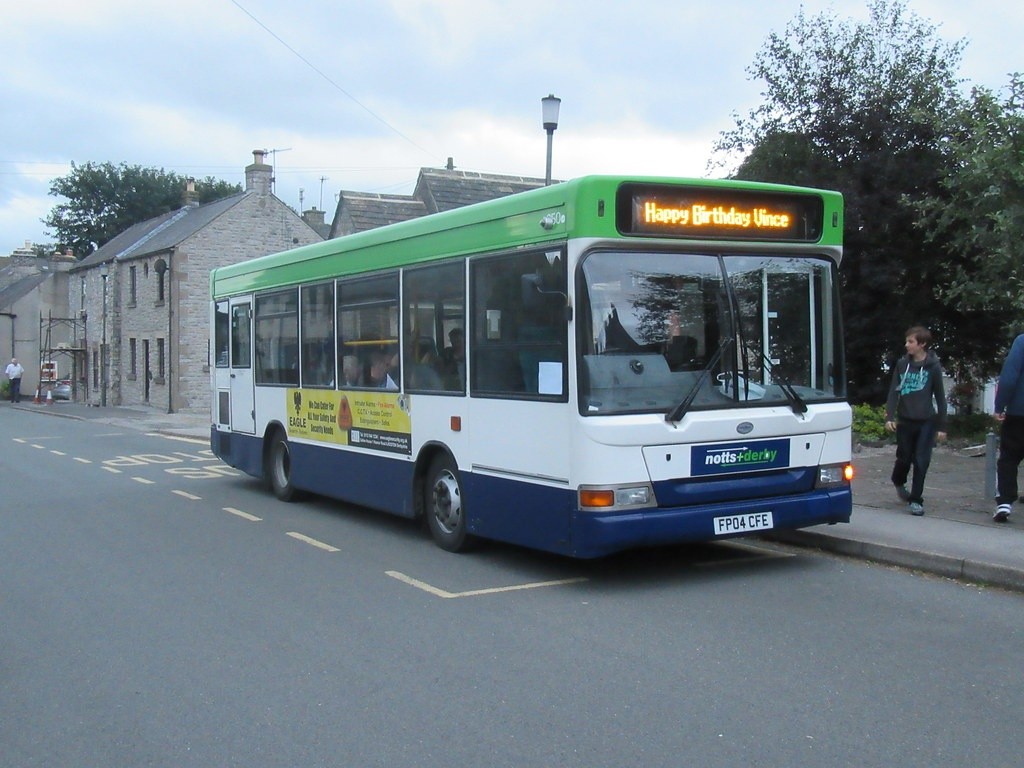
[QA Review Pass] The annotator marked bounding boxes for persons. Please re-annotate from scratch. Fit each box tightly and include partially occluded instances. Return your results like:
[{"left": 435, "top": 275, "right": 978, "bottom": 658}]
[
  {"left": 993, "top": 335, "right": 1024, "bottom": 520},
  {"left": 885, "top": 327, "right": 947, "bottom": 515},
  {"left": 598, "top": 270, "right": 683, "bottom": 370},
  {"left": 414, "top": 328, "right": 464, "bottom": 393},
  {"left": 330, "top": 328, "right": 399, "bottom": 389},
  {"left": 6, "top": 358, "right": 24, "bottom": 403}
]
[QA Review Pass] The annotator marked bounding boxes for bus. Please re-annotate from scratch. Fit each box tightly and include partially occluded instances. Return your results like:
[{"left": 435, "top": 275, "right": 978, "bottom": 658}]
[{"left": 207, "top": 173, "right": 852, "bottom": 559}]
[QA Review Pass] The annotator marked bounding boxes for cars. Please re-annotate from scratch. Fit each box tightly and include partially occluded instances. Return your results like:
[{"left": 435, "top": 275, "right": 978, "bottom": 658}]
[{"left": 51, "top": 381, "right": 71, "bottom": 400}]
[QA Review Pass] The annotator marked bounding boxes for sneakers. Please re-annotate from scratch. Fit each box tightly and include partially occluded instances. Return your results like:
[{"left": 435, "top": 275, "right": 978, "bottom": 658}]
[
  {"left": 910, "top": 502, "right": 923, "bottom": 515},
  {"left": 993, "top": 503, "right": 1011, "bottom": 522},
  {"left": 895, "top": 482, "right": 910, "bottom": 501}
]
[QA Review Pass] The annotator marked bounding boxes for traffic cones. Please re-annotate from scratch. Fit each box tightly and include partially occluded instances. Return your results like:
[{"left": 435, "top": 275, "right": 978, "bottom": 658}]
[
  {"left": 31, "top": 384, "right": 40, "bottom": 404},
  {"left": 46, "top": 387, "right": 53, "bottom": 404}
]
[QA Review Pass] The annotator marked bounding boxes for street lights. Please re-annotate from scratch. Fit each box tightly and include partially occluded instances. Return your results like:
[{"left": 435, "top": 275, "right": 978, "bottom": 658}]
[{"left": 540, "top": 93, "right": 562, "bottom": 184}]
